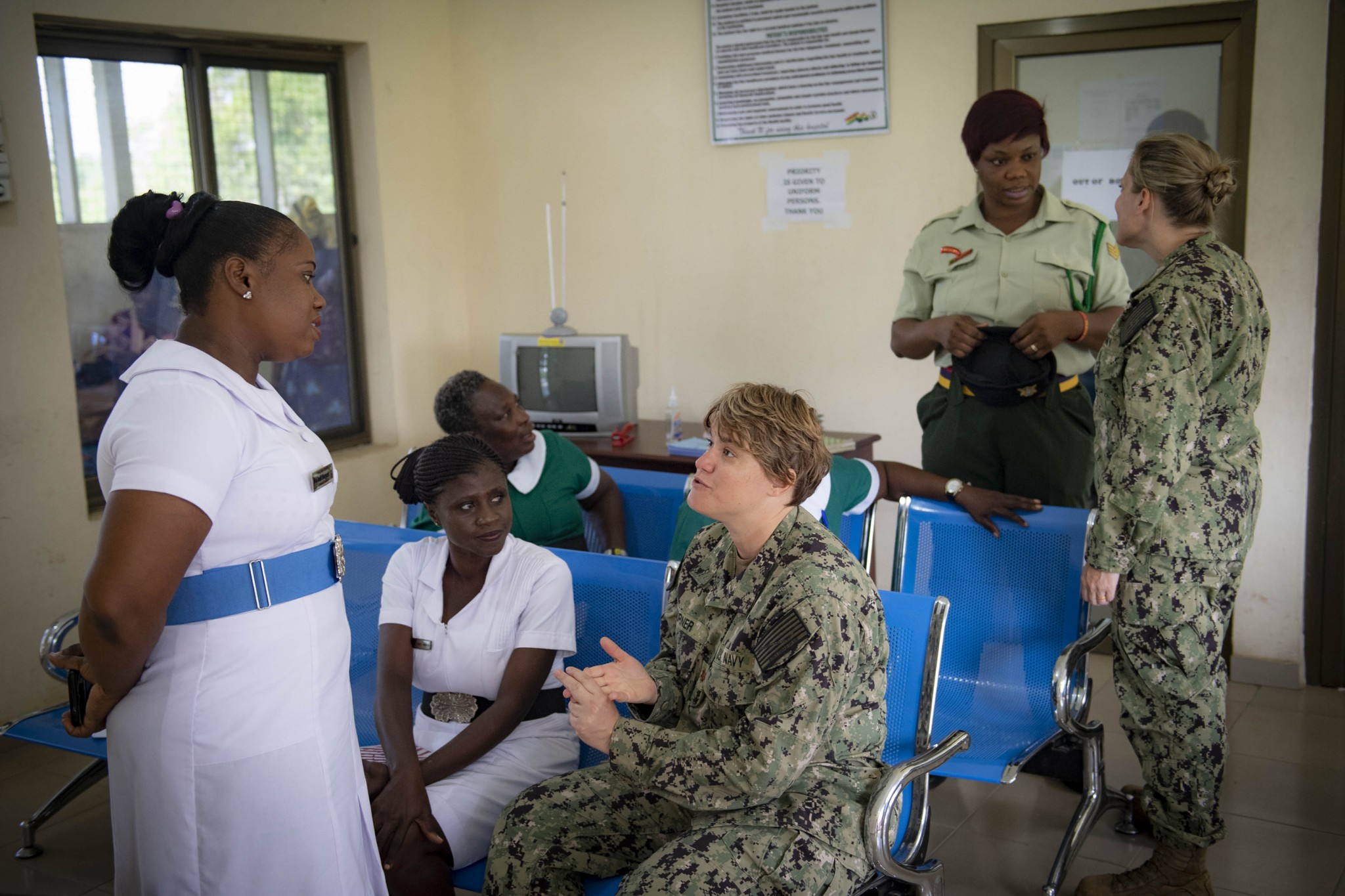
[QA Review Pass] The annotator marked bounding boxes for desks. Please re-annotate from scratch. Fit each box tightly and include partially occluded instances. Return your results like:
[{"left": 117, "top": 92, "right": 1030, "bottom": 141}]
[{"left": 570, "top": 420, "right": 880, "bottom": 474}]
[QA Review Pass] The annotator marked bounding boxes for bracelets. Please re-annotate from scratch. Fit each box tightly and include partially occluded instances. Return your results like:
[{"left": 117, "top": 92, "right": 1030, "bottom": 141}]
[{"left": 605, "top": 548, "right": 627, "bottom": 557}]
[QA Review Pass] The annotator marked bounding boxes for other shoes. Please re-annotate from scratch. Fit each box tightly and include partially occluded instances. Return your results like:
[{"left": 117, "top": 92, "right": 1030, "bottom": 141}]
[
  {"left": 1073, "top": 842, "right": 1215, "bottom": 896},
  {"left": 1121, "top": 784, "right": 1164, "bottom": 841}
]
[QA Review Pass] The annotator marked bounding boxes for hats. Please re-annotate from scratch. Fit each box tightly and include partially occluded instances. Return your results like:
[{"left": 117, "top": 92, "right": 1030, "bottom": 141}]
[{"left": 951, "top": 325, "right": 1059, "bottom": 407}]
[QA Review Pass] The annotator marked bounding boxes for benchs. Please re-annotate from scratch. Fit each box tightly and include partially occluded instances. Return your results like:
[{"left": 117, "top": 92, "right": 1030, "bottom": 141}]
[{"left": 0, "top": 467, "right": 1142, "bottom": 896}]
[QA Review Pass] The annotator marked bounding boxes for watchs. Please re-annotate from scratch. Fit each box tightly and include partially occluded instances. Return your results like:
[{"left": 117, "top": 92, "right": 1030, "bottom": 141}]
[{"left": 945, "top": 477, "right": 963, "bottom": 505}]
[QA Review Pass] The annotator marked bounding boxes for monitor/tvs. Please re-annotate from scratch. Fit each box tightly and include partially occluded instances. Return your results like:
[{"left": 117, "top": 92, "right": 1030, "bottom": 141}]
[{"left": 498, "top": 332, "right": 639, "bottom": 438}]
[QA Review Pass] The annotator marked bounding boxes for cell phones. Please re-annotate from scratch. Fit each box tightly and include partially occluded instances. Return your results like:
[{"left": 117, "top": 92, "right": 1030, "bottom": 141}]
[{"left": 68, "top": 654, "right": 86, "bottom": 728}]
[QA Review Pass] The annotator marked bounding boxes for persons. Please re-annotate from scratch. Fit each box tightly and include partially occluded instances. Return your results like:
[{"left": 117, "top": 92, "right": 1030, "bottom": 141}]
[
  {"left": 1082, "top": 132, "right": 1270, "bottom": 896},
  {"left": 361, "top": 438, "right": 584, "bottom": 895},
  {"left": 889, "top": 89, "right": 1136, "bottom": 792},
  {"left": 670, "top": 405, "right": 1043, "bottom": 571},
  {"left": 412, "top": 369, "right": 631, "bottom": 556},
  {"left": 486, "top": 382, "right": 889, "bottom": 896},
  {"left": 49, "top": 192, "right": 397, "bottom": 894}
]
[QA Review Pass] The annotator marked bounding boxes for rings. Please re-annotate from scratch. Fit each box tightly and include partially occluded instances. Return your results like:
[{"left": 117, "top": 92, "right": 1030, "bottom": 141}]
[{"left": 1031, "top": 345, "right": 1038, "bottom": 352}]
